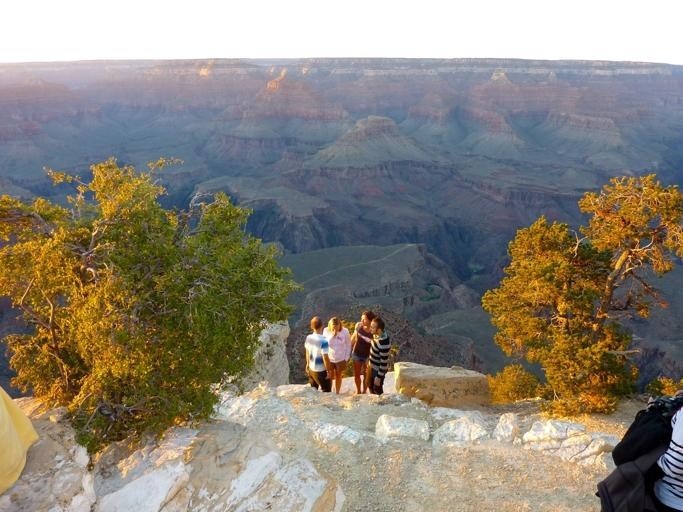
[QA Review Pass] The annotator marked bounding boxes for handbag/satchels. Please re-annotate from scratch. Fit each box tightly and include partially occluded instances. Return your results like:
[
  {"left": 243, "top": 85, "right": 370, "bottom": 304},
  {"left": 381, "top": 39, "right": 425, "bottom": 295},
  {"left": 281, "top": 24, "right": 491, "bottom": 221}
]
[{"left": 611, "top": 397, "right": 683, "bottom": 468}]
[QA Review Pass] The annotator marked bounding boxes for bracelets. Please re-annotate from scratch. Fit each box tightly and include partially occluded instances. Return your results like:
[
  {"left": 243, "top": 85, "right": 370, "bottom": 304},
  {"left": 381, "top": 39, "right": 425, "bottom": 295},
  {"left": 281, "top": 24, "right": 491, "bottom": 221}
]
[{"left": 325, "top": 369, "right": 332, "bottom": 373}]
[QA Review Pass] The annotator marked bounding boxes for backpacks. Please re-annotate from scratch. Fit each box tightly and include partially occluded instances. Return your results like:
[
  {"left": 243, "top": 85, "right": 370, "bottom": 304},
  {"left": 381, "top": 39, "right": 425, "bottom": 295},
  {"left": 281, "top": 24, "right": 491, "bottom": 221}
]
[{"left": 595, "top": 448, "right": 665, "bottom": 512}]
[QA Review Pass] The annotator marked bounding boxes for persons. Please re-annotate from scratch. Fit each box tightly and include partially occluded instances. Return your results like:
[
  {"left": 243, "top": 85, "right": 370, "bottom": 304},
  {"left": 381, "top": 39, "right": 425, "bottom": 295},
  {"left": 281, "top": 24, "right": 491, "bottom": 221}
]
[
  {"left": 348, "top": 309, "right": 378, "bottom": 395},
  {"left": 593, "top": 388, "right": 683, "bottom": 512},
  {"left": 322, "top": 316, "right": 352, "bottom": 394},
  {"left": 354, "top": 316, "right": 390, "bottom": 396},
  {"left": 302, "top": 315, "right": 332, "bottom": 392},
  {"left": 0, "top": 384, "right": 42, "bottom": 497}
]
